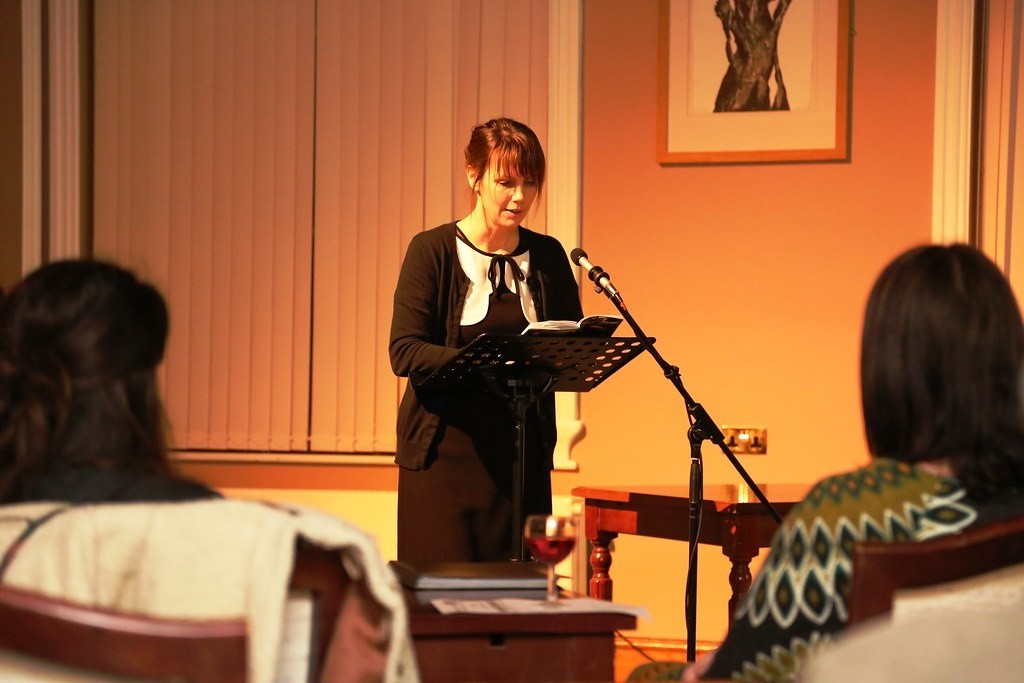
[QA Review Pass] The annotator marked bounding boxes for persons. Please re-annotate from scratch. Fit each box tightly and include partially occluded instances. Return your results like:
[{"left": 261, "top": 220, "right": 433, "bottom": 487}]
[
  {"left": 0, "top": 257, "right": 223, "bottom": 503},
  {"left": 387, "top": 117, "right": 585, "bottom": 561},
  {"left": 682, "top": 242, "right": 1023, "bottom": 682}
]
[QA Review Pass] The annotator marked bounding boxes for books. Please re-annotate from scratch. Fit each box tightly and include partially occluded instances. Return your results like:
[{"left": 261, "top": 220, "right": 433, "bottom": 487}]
[{"left": 505, "top": 314, "right": 623, "bottom": 366}]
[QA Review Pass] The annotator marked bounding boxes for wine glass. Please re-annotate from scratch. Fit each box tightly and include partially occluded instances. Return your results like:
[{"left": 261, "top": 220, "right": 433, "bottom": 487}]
[{"left": 524, "top": 516, "right": 580, "bottom": 608}]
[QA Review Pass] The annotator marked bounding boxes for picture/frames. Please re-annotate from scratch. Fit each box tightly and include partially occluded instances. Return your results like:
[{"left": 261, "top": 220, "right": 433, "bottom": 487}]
[{"left": 657, "top": 0, "right": 852, "bottom": 167}]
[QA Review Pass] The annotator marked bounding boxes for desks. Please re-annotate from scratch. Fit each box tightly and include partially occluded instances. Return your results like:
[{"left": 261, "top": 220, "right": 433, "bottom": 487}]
[{"left": 402, "top": 484, "right": 814, "bottom": 683}]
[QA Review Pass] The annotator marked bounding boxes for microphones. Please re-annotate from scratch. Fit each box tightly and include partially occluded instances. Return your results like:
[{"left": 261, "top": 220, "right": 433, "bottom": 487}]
[{"left": 571, "top": 247, "right": 623, "bottom": 305}]
